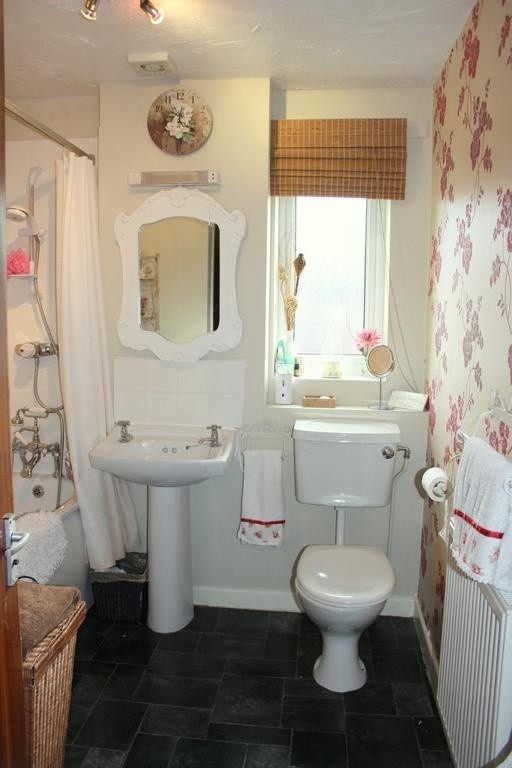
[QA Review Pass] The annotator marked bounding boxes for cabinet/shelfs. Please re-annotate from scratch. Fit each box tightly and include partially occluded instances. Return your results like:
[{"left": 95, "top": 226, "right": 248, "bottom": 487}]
[{"left": 22, "top": 601, "right": 87, "bottom": 768}]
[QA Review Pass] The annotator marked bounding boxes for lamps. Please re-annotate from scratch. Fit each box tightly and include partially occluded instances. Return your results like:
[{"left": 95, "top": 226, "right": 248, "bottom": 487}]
[
  {"left": 128, "top": 171, "right": 219, "bottom": 186},
  {"left": 80, "top": 0, "right": 100, "bottom": 22},
  {"left": 139, "top": 0, "right": 164, "bottom": 24}
]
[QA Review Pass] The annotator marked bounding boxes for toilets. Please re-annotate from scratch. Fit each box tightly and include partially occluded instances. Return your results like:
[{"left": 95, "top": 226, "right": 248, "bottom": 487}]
[{"left": 291, "top": 419, "right": 401, "bottom": 694}]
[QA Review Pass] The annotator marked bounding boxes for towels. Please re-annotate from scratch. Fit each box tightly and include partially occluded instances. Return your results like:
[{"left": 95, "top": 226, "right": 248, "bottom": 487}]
[
  {"left": 236, "top": 448, "right": 286, "bottom": 546},
  {"left": 437, "top": 436, "right": 512, "bottom": 592},
  {"left": 18, "top": 577, "right": 81, "bottom": 659}
]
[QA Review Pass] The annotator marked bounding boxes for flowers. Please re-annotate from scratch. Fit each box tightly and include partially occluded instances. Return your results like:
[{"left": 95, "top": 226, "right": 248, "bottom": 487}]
[{"left": 277, "top": 252, "right": 309, "bottom": 330}]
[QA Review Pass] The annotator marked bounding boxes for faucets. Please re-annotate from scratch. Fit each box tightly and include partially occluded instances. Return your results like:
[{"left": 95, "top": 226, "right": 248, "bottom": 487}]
[
  {"left": 117, "top": 421, "right": 134, "bottom": 443},
  {"left": 18, "top": 449, "right": 40, "bottom": 478},
  {"left": 199, "top": 425, "right": 222, "bottom": 447}
]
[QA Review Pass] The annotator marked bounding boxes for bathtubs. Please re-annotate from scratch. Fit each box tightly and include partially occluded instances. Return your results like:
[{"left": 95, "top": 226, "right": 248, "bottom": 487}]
[{"left": 12, "top": 473, "right": 95, "bottom": 613}]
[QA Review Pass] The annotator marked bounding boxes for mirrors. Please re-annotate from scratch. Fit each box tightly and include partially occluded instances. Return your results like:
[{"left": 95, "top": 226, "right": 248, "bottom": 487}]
[
  {"left": 366, "top": 345, "right": 395, "bottom": 410},
  {"left": 113, "top": 187, "right": 247, "bottom": 364}
]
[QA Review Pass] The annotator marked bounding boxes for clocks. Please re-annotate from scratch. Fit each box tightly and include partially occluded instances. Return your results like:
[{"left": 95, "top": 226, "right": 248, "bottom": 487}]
[{"left": 147, "top": 88, "right": 213, "bottom": 156}]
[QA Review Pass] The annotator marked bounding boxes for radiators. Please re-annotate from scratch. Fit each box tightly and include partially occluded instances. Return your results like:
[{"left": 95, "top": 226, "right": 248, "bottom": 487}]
[{"left": 435, "top": 552, "right": 512, "bottom": 768}]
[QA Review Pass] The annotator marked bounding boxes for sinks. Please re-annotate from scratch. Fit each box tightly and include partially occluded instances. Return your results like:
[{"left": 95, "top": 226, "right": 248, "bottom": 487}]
[{"left": 89, "top": 423, "right": 235, "bottom": 487}]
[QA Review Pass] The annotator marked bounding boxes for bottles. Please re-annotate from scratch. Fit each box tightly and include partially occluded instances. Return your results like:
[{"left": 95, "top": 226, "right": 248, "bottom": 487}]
[{"left": 275, "top": 364, "right": 292, "bottom": 404}]
